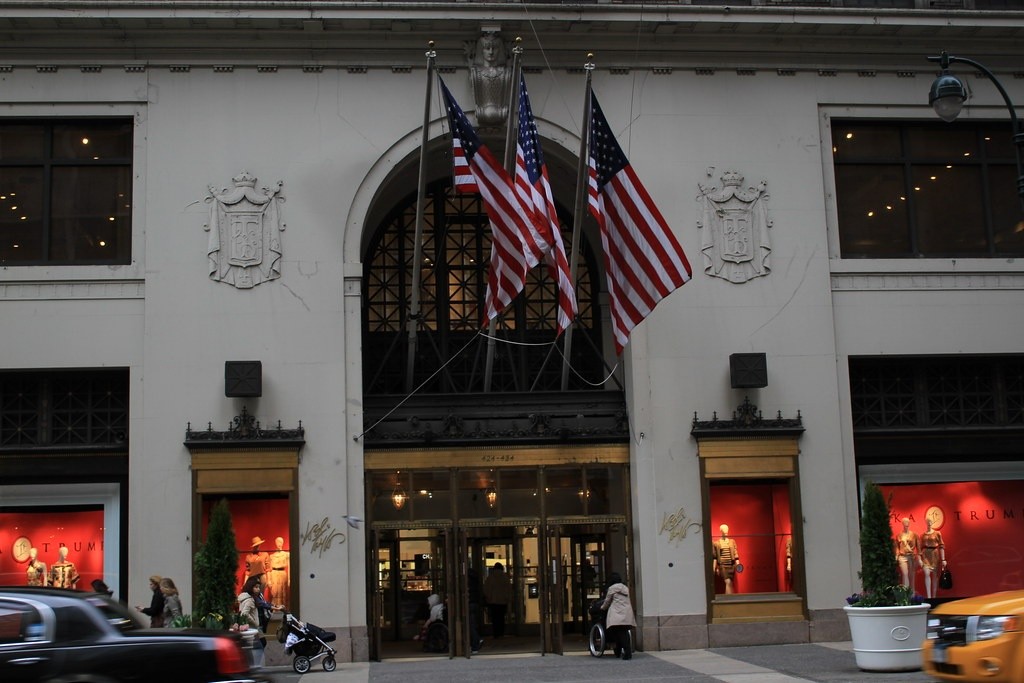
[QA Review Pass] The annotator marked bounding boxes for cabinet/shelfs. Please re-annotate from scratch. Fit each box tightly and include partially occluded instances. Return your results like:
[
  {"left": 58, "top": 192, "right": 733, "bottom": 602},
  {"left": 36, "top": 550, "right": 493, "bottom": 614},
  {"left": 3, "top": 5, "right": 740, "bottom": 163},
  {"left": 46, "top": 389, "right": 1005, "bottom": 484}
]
[{"left": 377, "top": 559, "right": 416, "bottom": 580}]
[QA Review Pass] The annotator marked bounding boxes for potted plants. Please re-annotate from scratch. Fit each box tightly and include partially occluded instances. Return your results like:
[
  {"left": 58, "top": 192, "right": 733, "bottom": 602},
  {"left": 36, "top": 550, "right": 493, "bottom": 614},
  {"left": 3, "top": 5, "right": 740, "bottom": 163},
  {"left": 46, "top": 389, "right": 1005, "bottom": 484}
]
[
  {"left": 843, "top": 477, "right": 931, "bottom": 672},
  {"left": 170, "top": 495, "right": 266, "bottom": 673}
]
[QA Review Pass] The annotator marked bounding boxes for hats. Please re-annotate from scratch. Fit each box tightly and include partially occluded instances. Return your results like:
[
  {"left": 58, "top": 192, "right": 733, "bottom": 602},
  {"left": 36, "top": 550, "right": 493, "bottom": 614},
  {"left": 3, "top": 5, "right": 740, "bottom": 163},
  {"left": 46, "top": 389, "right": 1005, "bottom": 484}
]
[{"left": 250, "top": 536, "right": 265, "bottom": 548}]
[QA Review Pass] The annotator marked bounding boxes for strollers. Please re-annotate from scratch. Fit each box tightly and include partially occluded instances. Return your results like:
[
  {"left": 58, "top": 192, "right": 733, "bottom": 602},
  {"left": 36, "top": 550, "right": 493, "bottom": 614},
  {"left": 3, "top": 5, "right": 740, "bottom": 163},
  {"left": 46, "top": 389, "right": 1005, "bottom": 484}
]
[{"left": 279, "top": 608, "right": 338, "bottom": 674}]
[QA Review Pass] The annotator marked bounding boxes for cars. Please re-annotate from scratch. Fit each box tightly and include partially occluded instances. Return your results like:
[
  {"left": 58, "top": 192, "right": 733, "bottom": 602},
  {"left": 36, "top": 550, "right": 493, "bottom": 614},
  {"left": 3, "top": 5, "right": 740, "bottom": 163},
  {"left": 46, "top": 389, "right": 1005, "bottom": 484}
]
[{"left": 0, "top": 586, "right": 275, "bottom": 683}]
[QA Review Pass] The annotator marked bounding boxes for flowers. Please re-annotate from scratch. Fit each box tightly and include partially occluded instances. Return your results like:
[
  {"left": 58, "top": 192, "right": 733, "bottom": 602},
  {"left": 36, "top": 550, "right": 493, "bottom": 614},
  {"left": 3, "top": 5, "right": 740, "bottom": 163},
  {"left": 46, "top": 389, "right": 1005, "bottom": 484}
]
[
  {"left": 170, "top": 611, "right": 252, "bottom": 632},
  {"left": 844, "top": 585, "right": 926, "bottom": 606}
]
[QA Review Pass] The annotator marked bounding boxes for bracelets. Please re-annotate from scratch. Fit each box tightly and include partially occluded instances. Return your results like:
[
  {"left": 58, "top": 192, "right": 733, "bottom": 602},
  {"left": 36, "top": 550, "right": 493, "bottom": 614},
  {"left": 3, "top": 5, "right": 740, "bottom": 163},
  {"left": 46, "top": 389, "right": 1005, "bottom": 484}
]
[{"left": 942, "top": 559, "right": 945, "bottom": 561}]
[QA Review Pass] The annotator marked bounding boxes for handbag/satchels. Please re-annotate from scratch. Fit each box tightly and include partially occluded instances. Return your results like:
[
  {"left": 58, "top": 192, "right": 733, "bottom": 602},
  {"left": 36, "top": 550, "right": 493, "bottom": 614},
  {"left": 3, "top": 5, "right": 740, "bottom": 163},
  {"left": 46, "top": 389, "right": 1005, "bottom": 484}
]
[
  {"left": 939, "top": 565, "right": 953, "bottom": 590},
  {"left": 277, "top": 613, "right": 288, "bottom": 644}
]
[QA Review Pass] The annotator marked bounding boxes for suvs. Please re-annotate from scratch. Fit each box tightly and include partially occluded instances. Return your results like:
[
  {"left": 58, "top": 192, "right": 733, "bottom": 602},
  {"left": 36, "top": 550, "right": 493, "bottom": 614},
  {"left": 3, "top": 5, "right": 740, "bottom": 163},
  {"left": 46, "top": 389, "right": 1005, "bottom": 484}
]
[{"left": 922, "top": 589, "right": 1024, "bottom": 683}]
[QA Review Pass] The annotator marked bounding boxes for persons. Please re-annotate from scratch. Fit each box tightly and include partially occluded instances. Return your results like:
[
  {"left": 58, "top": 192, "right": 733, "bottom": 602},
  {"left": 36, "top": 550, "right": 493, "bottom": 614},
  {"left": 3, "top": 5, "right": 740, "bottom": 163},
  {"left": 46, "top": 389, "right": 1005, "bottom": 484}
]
[
  {"left": 414, "top": 563, "right": 512, "bottom": 655},
  {"left": 895, "top": 517, "right": 922, "bottom": 597},
  {"left": 242, "top": 536, "right": 272, "bottom": 604},
  {"left": 137, "top": 576, "right": 165, "bottom": 628},
  {"left": 49, "top": 547, "right": 80, "bottom": 589},
  {"left": 712, "top": 524, "right": 743, "bottom": 595},
  {"left": 786, "top": 524, "right": 793, "bottom": 572},
  {"left": 267, "top": 537, "right": 290, "bottom": 611},
  {"left": 237, "top": 578, "right": 266, "bottom": 667},
  {"left": 91, "top": 579, "right": 113, "bottom": 598},
  {"left": 920, "top": 516, "right": 948, "bottom": 599},
  {"left": 27, "top": 547, "right": 47, "bottom": 587},
  {"left": 585, "top": 559, "right": 638, "bottom": 660},
  {"left": 160, "top": 578, "right": 183, "bottom": 628},
  {"left": 254, "top": 576, "right": 286, "bottom": 635},
  {"left": 471, "top": 37, "right": 511, "bottom": 121}
]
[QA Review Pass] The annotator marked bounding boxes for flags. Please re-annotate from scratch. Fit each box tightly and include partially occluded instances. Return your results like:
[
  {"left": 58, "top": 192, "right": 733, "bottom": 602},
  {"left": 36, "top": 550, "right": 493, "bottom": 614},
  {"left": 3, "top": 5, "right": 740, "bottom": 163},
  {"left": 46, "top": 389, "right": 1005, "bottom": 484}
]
[
  {"left": 437, "top": 74, "right": 557, "bottom": 326},
  {"left": 516, "top": 68, "right": 578, "bottom": 341},
  {"left": 587, "top": 89, "right": 691, "bottom": 360}
]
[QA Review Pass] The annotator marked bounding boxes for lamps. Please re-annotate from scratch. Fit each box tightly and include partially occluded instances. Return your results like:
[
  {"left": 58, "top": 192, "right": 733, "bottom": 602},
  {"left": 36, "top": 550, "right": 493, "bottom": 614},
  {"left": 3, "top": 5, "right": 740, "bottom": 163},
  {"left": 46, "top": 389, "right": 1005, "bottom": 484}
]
[
  {"left": 391, "top": 468, "right": 408, "bottom": 512},
  {"left": 485, "top": 466, "right": 498, "bottom": 513}
]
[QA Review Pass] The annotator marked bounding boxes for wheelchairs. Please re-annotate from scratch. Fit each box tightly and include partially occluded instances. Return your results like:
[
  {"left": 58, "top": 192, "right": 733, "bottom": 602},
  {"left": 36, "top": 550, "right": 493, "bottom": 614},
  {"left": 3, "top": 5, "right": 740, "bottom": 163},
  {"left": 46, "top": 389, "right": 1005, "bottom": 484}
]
[{"left": 589, "top": 622, "right": 634, "bottom": 658}]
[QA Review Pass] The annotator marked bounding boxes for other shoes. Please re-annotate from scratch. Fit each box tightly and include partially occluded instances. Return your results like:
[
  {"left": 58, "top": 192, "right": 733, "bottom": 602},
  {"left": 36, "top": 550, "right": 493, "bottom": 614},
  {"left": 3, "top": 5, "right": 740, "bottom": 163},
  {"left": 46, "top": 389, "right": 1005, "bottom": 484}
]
[
  {"left": 413, "top": 635, "right": 420, "bottom": 639},
  {"left": 614, "top": 648, "right": 621, "bottom": 657},
  {"left": 470, "top": 649, "right": 478, "bottom": 654},
  {"left": 622, "top": 654, "right": 632, "bottom": 660}
]
